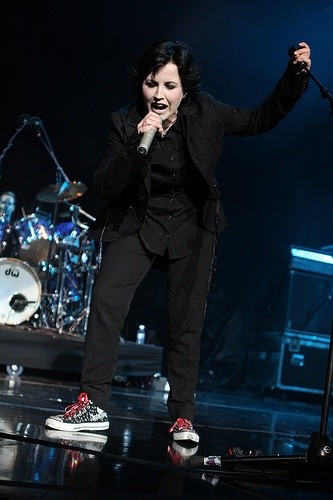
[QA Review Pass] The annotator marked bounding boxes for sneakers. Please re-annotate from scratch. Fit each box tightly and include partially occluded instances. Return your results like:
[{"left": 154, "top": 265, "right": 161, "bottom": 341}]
[
  {"left": 46, "top": 392, "right": 110, "bottom": 431},
  {"left": 168, "top": 417, "right": 200, "bottom": 443}
]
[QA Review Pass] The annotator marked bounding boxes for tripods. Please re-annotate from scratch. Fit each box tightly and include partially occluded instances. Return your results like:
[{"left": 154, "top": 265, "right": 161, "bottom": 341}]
[{"left": 27, "top": 199, "right": 126, "bottom": 342}]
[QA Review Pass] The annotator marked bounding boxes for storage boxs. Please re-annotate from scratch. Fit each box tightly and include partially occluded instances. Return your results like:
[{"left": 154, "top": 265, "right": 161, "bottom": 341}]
[{"left": 247, "top": 330, "right": 330, "bottom": 396}]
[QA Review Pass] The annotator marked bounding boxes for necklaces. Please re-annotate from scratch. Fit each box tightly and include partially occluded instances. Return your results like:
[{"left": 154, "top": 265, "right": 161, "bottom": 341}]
[{"left": 161, "top": 121, "right": 174, "bottom": 137}]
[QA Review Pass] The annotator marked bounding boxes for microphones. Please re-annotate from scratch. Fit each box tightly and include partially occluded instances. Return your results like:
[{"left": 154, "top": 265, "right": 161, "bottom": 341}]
[{"left": 137, "top": 116, "right": 162, "bottom": 155}]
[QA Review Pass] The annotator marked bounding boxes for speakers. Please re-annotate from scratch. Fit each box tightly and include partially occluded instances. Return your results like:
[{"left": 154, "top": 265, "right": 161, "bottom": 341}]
[{"left": 255, "top": 269, "right": 333, "bottom": 338}]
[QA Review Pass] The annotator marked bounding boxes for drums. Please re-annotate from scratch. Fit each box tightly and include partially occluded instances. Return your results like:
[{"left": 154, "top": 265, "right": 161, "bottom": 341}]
[
  {"left": 0, "top": 257, "right": 42, "bottom": 326},
  {"left": 8, "top": 213, "right": 58, "bottom": 263},
  {"left": 74, "top": 246, "right": 98, "bottom": 273},
  {"left": 56, "top": 221, "right": 93, "bottom": 251},
  {"left": 28, "top": 258, "right": 56, "bottom": 281}
]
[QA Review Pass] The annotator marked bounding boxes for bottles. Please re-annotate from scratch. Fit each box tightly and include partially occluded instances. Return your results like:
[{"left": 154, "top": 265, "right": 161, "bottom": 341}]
[{"left": 137, "top": 325, "right": 145, "bottom": 345}]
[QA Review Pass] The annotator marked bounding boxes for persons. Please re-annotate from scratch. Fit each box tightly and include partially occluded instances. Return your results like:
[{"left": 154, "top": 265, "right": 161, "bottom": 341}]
[{"left": 45, "top": 40, "right": 312, "bottom": 444}]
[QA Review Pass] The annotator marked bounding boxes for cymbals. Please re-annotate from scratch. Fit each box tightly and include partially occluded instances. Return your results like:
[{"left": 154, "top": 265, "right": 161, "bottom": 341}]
[{"left": 36, "top": 181, "right": 86, "bottom": 202}]
